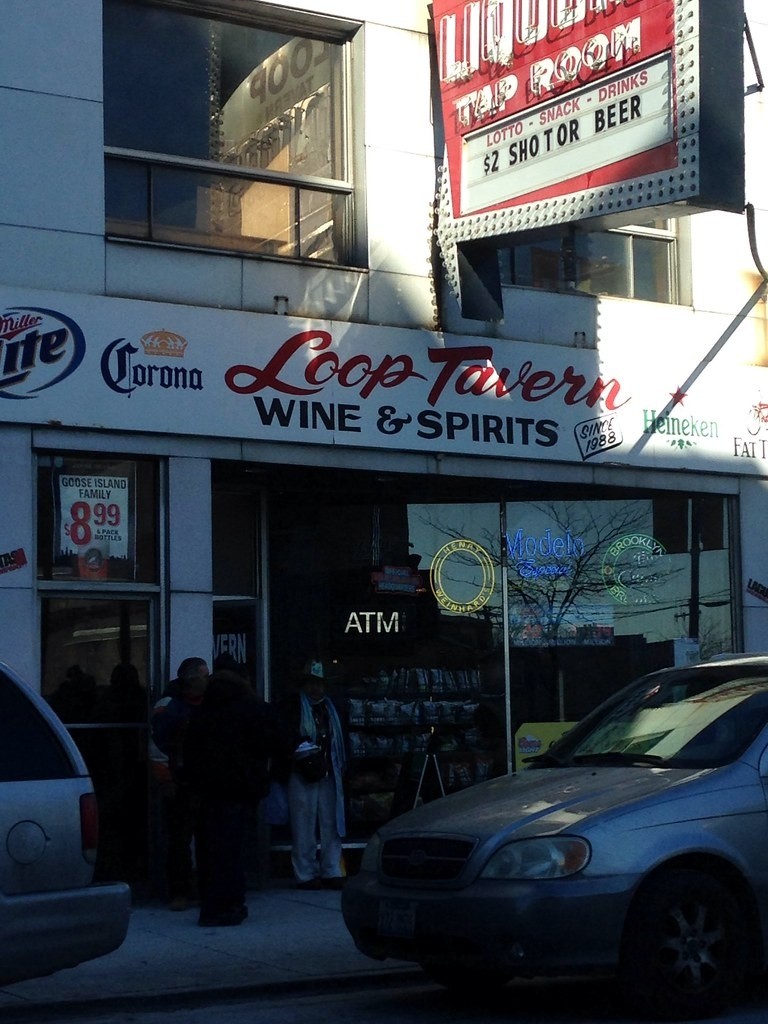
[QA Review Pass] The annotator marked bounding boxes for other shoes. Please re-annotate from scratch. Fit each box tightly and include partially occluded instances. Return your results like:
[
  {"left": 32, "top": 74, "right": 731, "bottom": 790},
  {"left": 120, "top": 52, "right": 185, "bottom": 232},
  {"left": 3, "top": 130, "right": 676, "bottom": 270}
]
[
  {"left": 171, "top": 900, "right": 186, "bottom": 909},
  {"left": 297, "top": 878, "right": 321, "bottom": 888},
  {"left": 320, "top": 876, "right": 342, "bottom": 890},
  {"left": 198, "top": 904, "right": 248, "bottom": 926},
  {"left": 187, "top": 899, "right": 199, "bottom": 905}
]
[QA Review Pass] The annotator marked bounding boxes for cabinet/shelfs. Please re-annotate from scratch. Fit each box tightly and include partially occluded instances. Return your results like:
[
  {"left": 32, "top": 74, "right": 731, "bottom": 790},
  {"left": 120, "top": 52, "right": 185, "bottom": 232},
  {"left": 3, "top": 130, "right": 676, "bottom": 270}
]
[{"left": 338, "top": 689, "right": 507, "bottom": 849}]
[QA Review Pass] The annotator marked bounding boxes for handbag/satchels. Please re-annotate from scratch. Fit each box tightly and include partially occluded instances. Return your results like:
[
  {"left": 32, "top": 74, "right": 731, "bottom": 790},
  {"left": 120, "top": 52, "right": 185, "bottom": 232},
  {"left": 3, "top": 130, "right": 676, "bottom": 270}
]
[{"left": 299, "top": 752, "right": 326, "bottom": 783}]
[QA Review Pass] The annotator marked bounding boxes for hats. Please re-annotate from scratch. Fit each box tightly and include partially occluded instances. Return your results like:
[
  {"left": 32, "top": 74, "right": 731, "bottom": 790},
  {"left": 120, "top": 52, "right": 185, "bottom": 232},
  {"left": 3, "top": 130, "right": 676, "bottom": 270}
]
[
  {"left": 297, "top": 662, "right": 326, "bottom": 681},
  {"left": 213, "top": 653, "right": 249, "bottom": 674}
]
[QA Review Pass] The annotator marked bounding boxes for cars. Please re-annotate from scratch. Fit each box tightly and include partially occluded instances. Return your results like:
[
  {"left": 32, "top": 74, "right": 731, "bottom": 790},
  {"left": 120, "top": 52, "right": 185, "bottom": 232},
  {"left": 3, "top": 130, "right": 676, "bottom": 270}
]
[
  {"left": 342, "top": 652, "right": 768, "bottom": 1023},
  {"left": 1, "top": 660, "right": 133, "bottom": 987}
]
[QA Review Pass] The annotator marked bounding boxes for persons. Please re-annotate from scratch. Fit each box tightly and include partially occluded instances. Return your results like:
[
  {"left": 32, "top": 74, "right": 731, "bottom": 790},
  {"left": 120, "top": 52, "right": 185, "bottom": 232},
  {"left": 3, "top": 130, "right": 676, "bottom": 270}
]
[
  {"left": 143, "top": 651, "right": 277, "bottom": 927},
  {"left": 275, "top": 659, "right": 353, "bottom": 891},
  {"left": 46, "top": 663, "right": 160, "bottom": 883}
]
